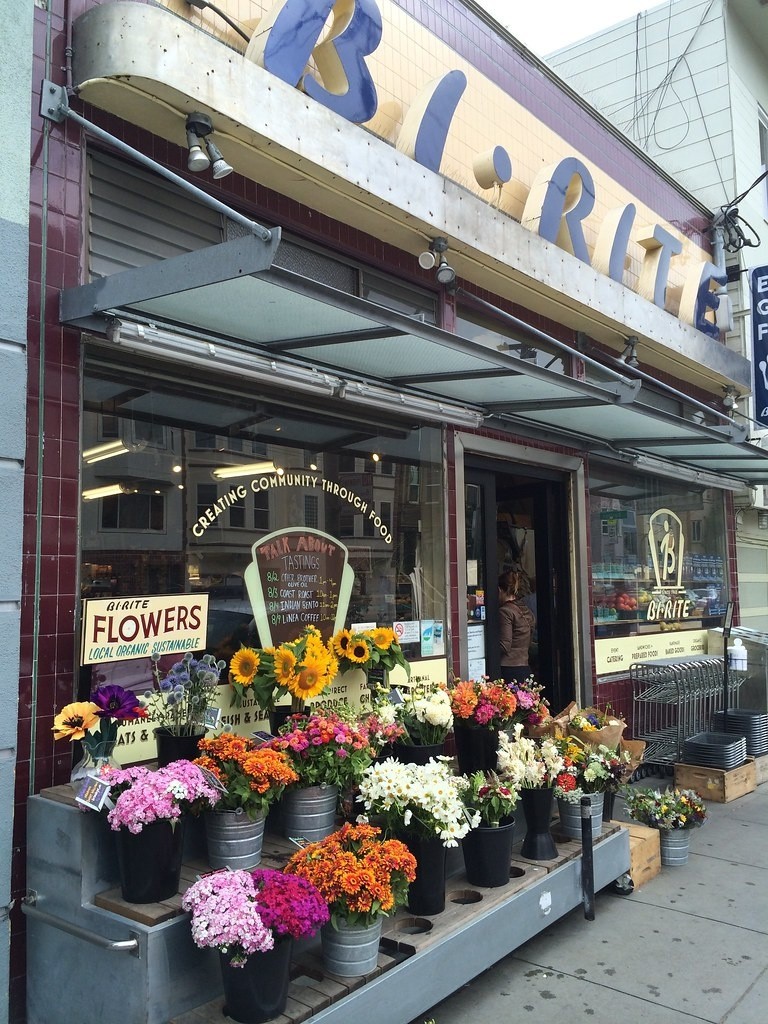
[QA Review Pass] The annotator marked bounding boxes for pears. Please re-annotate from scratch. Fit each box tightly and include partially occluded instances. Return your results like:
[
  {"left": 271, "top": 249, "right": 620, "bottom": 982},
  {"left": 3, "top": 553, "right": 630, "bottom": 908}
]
[{"left": 635, "top": 590, "right": 652, "bottom": 602}]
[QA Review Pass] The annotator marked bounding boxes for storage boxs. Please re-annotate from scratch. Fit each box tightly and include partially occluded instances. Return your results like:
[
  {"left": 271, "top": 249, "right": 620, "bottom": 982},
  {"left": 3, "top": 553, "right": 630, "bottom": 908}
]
[
  {"left": 675, "top": 758, "right": 757, "bottom": 803},
  {"left": 609, "top": 819, "right": 661, "bottom": 890}
]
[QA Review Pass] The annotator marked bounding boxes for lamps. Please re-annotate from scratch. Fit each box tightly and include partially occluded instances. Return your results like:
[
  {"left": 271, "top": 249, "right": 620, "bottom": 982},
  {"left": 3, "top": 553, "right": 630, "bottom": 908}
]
[
  {"left": 186, "top": 112, "right": 234, "bottom": 179},
  {"left": 419, "top": 237, "right": 455, "bottom": 284},
  {"left": 82, "top": 437, "right": 145, "bottom": 466},
  {"left": 82, "top": 480, "right": 137, "bottom": 501},
  {"left": 211, "top": 458, "right": 280, "bottom": 481},
  {"left": 615, "top": 336, "right": 643, "bottom": 369},
  {"left": 723, "top": 385, "right": 741, "bottom": 408}
]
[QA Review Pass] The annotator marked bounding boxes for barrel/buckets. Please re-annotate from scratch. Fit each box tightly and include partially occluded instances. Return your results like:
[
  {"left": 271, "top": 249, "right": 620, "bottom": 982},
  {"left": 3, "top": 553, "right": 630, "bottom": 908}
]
[{"left": 118, "top": 716, "right": 689, "bottom": 1024}]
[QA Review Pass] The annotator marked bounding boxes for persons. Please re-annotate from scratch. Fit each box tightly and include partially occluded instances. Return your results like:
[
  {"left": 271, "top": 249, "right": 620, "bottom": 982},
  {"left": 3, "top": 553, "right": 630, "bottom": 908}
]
[{"left": 488, "top": 570, "right": 535, "bottom": 686}]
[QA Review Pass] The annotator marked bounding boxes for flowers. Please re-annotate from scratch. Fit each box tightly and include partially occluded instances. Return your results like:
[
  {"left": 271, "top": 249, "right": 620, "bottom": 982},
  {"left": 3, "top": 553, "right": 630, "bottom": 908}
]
[{"left": 53, "top": 627, "right": 704, "bottom": 970}]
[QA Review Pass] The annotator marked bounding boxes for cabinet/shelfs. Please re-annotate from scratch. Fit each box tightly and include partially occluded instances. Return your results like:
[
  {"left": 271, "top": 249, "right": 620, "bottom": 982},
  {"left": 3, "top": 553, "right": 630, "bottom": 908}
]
[{"left": 590, "top": 578, "right": 723, "bottom": 625}]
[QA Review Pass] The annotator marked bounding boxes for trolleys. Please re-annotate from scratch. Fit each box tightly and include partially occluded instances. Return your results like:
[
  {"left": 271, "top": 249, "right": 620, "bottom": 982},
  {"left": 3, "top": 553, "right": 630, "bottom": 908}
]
[{"left": 625, "top": 653, "right": 752, "bottom": 784}]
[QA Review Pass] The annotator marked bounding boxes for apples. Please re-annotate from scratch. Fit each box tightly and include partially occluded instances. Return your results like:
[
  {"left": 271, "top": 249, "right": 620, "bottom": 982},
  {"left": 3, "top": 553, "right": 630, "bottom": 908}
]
[{"left": 600, "top": 593, "right": 638, "bottom": 611}]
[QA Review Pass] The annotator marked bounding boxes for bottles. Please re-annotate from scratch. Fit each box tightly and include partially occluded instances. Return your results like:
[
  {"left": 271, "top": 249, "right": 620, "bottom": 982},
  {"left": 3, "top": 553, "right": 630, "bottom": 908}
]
[{"left": 682, "top": 554, "right": 722, "bottom": 590}]
